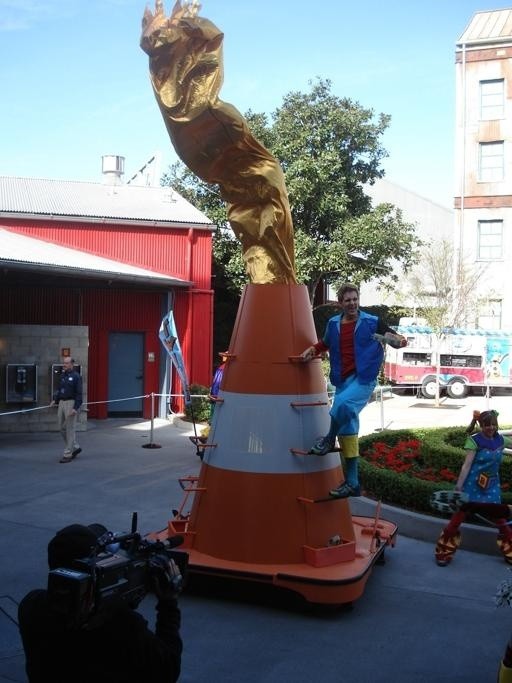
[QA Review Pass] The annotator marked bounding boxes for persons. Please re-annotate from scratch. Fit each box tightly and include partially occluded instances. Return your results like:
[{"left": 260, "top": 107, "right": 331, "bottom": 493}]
[
  {"left": 433, "top": 409, "right": 511, "bottom": 567},
  {"left": 299, "top": 278, "right": 408, "bottom": 500},
  {"left": 49, "top": 356, "right": 83, "bottom": 464},
  {"left": 18, "top": 522, "right": 184, "bottom": 682},
  {"left": 199, "top": 348, "right": 230, "bottom": 445}
]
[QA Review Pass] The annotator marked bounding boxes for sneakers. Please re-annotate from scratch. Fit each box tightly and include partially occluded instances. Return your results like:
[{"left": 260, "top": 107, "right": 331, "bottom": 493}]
[
  {"left": 59, "top": 447, "right": 82, "bottom": 464},
  {"left": 311, "top": 437, "right": 336, "bottom": 456},
  {"left": 329, "top": 482, "right": 361, "bottom": 498},
  {"left": 437, "top": 558, "right": 450, "bottom": 567}
]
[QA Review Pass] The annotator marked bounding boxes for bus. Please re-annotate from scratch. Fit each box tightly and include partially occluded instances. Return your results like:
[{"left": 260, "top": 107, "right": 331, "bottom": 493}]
[{"left": 382, "top": 327, "right": 512, "bottom": 399}]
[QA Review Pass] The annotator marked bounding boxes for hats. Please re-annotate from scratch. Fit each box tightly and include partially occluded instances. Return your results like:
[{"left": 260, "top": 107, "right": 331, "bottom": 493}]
[{"left": 48, "top": 524, "right": 109, "bottom": 569}]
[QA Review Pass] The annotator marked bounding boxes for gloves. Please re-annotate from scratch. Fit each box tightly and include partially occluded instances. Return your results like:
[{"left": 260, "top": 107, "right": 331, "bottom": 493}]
[{"left": 301, "top": 346, "right": 317, "bottom": 363}]
